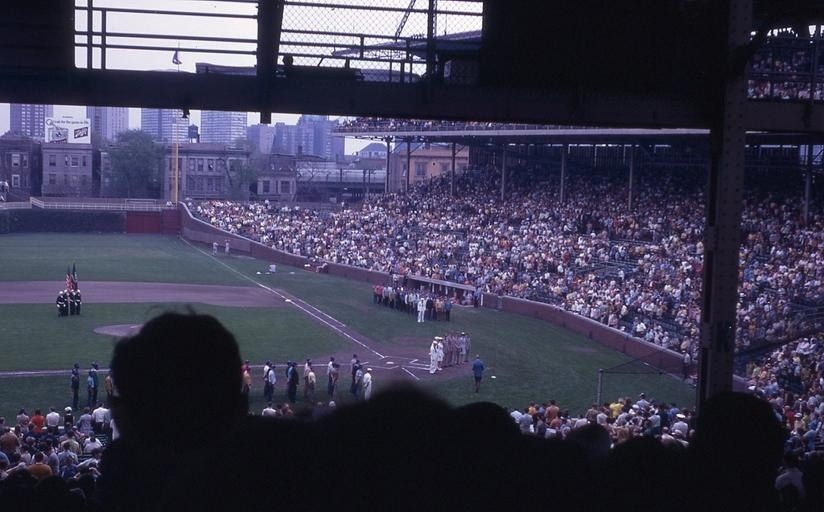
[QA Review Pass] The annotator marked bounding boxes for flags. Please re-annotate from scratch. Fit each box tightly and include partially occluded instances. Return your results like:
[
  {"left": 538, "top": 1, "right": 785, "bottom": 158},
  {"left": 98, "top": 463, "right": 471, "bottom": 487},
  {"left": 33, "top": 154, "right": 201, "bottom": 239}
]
[{"left": 65, "top": 263, "right": 78, "bottom": 291}]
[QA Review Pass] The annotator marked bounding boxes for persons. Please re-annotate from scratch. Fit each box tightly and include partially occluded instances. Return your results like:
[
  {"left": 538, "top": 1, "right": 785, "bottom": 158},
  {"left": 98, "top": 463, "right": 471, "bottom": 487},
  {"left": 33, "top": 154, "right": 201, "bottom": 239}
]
[
  {"left": 749, "top": 30, "right": 823, "bottom": 100},
  {"left": 0, "top": 354, "right": 824, "bottom": 512},
  {"left": 166, "top": 155, "right": 823, "bottom": 372},
  {"left": 103, "top": 308, "right": 250, "bottom": 512},
  {"left": 56, "top": 289, "right": 82, "bottom": 317},
  {"left": 333, "top": 114, "right": 494, "bottom": 133}
]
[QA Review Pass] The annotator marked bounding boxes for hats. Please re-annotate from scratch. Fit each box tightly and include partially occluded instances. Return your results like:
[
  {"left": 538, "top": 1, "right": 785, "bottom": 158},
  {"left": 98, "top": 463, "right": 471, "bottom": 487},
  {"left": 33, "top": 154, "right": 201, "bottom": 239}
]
[
  {"left": 59, "top": 288, "right": 80, "bottom": 297},
  {"left": 373, "top": 271, "right": 563, "bottom": 304},
  {"left": 564, "top": 272, "right": 702, "bottom": 354},
  {"left": 513, "top": 392, "right": 695, "bottom": 442},
  {"left": 241, "top": 351, "right": 373, "bottom": 382},
  {"left": 186, "top": 198, "right": 705, "bottom": 271},
  {"left": 432, "top": 330, "right": 469, "bottom": 344},
  {"left": 0, "top": 361, "right": 113, "bottom": 479},
  {"left": 736, "top": 196, "right": 824, "bottom": 454}
]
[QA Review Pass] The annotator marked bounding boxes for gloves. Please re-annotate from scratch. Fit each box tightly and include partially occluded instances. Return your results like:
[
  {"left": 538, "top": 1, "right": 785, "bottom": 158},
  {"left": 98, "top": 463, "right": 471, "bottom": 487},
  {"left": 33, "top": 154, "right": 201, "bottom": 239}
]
[{"left": 57, "top": 299, "right": 82, "bottom": 308}]
